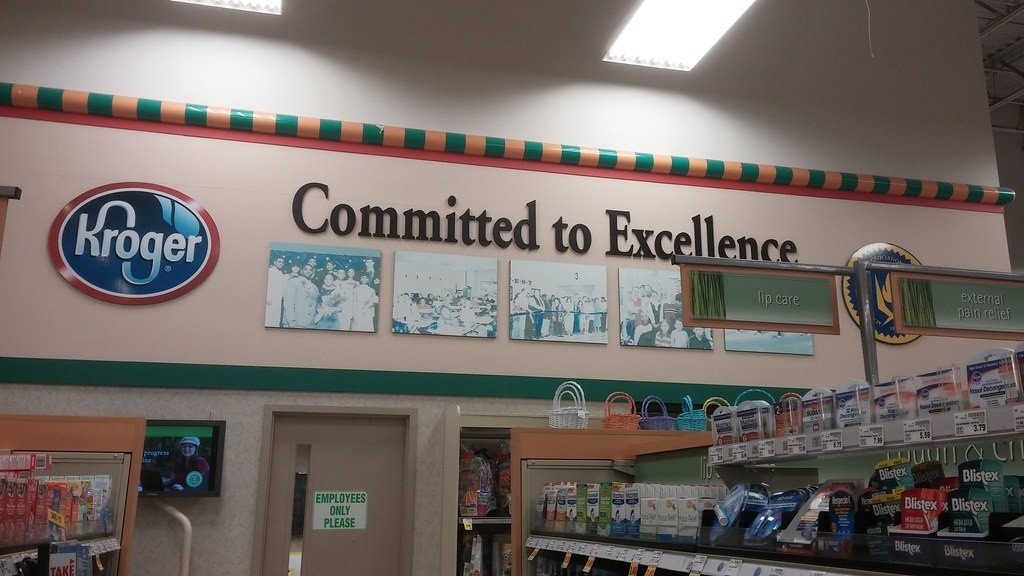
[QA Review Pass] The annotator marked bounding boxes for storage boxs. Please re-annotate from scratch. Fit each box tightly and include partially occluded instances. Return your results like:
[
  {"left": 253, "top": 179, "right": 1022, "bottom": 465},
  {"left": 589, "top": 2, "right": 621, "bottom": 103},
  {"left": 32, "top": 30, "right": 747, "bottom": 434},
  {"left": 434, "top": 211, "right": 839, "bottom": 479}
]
[{"left": 534, "top": 482, "right": 725, "bottom": 542}]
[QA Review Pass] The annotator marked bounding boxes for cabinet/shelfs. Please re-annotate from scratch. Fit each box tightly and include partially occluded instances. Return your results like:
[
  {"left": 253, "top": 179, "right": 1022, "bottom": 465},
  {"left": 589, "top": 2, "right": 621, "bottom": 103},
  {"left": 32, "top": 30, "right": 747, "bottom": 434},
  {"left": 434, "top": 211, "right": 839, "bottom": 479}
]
[
  {"left": 0, "top": 414, "right": 146, "bottom": 575},
  {"left": 712, "top": 396, "right": 1024, "bottom": 574},
  {"left": 443, "top": 410, "right": 512, "bottom": 576},
  {"left": 509, "top": 412, "right": 711, "bottom": 576}
]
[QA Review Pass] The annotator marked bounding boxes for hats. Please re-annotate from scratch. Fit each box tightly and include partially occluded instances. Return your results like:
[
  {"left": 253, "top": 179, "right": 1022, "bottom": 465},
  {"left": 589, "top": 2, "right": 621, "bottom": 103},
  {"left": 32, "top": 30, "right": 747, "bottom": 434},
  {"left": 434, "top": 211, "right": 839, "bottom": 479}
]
[{"left": 180, "top": 436, "right": 200, "bottom": 446}]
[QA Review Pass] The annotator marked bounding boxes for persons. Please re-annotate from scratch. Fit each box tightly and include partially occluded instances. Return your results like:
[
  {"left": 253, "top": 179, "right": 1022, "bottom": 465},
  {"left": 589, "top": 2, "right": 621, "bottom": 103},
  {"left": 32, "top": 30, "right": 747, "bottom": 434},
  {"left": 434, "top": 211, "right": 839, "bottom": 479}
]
[
  {"left": 265, "top": 255, "right": 379, "bottom": 333},
  {"left": 164, "top": 436, "right": 209, "bottom": 491},
  {"left": 393, "top": 287, "right": 498, "bottom": 338},
  {"left": 620, "top": 279, "right": 713, "bottom": 349},
  {"left": 512, "top": 287, "right": 608, "bottom": 340}
]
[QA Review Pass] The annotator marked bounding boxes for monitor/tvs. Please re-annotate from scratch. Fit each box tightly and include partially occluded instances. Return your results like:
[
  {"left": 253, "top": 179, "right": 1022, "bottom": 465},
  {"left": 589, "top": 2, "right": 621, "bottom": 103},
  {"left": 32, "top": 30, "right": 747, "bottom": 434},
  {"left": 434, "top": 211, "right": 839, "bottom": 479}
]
[{"left": 138, "top": 420, "right": 226, "bottom": 497}]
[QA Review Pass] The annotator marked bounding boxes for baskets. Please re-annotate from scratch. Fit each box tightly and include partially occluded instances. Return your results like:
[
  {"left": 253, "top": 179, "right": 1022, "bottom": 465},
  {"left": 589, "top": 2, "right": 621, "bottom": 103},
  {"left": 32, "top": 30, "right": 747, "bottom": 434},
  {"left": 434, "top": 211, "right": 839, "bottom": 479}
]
[{"left": 549, "top": 381, "right": 804, "bottom": 432}]
